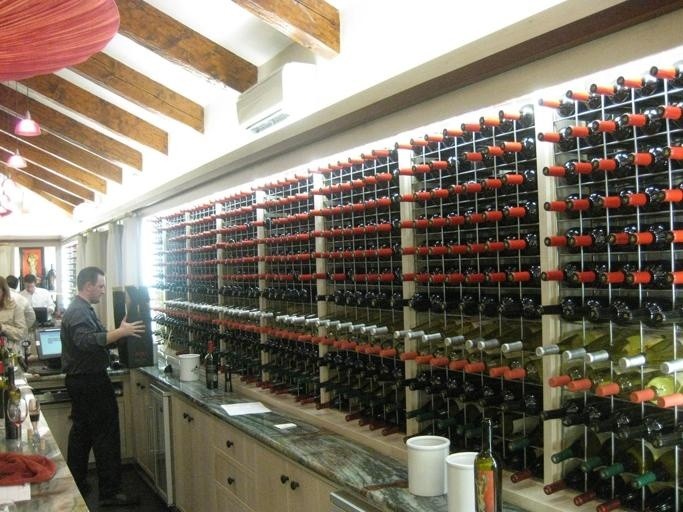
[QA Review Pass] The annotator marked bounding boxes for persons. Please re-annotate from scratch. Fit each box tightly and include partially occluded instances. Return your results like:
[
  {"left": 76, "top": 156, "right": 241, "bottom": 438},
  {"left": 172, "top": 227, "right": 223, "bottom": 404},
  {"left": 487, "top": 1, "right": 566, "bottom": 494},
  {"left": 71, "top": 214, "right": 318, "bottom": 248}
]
[
  {"left": 16, "top": 274, "right": 56, "bottom": 321},
  {"left": 60, "top": 266, "right": 146, "bottom": 507},
  {"left": 0, "top": 277, "right": 27, "bottom": 357},
  {"left": 5, "top": 275, "right": 36, "bottom": 332}
]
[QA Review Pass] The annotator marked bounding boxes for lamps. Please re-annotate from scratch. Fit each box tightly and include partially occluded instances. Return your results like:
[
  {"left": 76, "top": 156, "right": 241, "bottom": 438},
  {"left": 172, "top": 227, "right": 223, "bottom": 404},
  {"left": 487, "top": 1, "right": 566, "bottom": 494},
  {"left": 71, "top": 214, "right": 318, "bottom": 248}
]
[
  {"left": 15, "top": 75, "right": 44, "bottom": 143},
  {"left": 7, "top": 81, "right": 31, "bottom": 176}
]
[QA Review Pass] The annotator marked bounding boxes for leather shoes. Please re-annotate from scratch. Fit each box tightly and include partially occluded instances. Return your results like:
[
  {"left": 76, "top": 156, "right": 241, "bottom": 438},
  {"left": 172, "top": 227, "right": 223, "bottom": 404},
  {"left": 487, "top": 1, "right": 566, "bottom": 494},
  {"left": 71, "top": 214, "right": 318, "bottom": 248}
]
[{"left": 98, "top": 492, "right": 139, "bottom": 506}]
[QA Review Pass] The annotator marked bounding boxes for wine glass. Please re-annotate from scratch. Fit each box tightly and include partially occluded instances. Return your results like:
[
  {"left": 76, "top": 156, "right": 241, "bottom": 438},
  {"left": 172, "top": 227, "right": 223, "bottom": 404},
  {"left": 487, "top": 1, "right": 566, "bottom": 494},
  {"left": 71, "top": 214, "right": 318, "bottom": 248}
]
[
  {"left": 28, "top": 399, "right": 39, "bottom": 431},
  {"left": 6, "top": 398, "right": 28, "bottom": 448}
]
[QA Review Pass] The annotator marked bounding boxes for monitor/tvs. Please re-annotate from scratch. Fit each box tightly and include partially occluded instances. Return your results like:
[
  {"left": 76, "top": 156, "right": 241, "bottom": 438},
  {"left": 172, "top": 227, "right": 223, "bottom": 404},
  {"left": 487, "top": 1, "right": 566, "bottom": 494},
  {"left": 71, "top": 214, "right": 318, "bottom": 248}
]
[{"left": 35, "top": 328, "right": 62, "bottom": 370}]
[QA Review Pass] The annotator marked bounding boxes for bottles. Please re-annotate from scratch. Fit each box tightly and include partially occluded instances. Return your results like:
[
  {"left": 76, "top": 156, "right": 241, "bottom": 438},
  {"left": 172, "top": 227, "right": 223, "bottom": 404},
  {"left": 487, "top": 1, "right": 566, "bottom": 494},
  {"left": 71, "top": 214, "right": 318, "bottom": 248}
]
[
  {"left": 165, "top": 338, "right": 206, "bottom": 355},
  {"left": 401, "top": 318, "right": 480, "bottom": 450},
  {"left": 206, "top": 307, "right": 218, "bottom": 339},
  {"left": 21, "top": 428, "right": 45, "bottom": 463},
  {"left": 155, "top": 339, "right": 166, "bottom": 370},
  {"left": 249, "top": 172, "right": 316, "bottom": 304},
  {"left": 390, "top": 103, "right": 537, "bottom": 320},
  {"left": 149, "top": 208, "right": 191, "bottom": 300},
  {"left": 203, "top": 340, "right": 217, "bottom": 389},
  {"left": 2, "top": 366, "right": 20, "bottom": 439},
  {"left": 536, "top": 67, "right": 683, "bottom": 326},
  {"left": 0, "top": 361, "right": 6, "bottom": 418},
  {"left": 476, "top": 319, "right": 498, "bottom": 416},
  {"left": 535, "top": 326, "right": 683, "bottom": 512},
  {"left": 178, "top": 200, "right": 220, "bottom": 300},
  {"left": 0, "top": 334, "right": 20, "bottom": 366},
  {"left": 150, "top": 307, "right": 206, "bottom": 338},
  {"left": 208, "top": 188, "right": 262, "bottom": 300},
  {"left": 309, "top": 143, "right": 389, "bottom": 309},
  {"left": 497, "top": 320, "right": 535, "bottom": 482},
  {"left": 218, "top": 308, "right": 401, "bottom": 437},
  {"left": 473, "top": 418, "right": 502, "bottom": 512}
]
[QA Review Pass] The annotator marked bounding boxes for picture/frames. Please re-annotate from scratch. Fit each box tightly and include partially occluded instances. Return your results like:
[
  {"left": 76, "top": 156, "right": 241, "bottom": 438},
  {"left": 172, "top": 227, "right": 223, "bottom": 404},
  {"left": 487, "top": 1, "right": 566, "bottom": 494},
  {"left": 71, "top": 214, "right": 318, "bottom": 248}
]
[{"left": 17, "top": 246, "right": 45, "bottom": 292}]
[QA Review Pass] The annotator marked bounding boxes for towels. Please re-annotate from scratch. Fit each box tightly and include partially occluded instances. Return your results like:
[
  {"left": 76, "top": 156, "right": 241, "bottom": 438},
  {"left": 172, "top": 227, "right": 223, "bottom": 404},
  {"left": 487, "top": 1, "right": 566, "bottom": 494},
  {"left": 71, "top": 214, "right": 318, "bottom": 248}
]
[{"left": 2, "top": 451, "right": 56, "bottom": 486}]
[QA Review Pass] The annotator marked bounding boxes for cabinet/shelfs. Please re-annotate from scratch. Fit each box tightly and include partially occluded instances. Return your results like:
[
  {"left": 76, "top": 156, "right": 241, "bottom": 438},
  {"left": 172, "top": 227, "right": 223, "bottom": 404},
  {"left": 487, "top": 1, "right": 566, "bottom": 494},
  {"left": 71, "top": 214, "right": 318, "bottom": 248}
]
[{"left": 29, "top": 374, "right": 131, "bottom": 466}]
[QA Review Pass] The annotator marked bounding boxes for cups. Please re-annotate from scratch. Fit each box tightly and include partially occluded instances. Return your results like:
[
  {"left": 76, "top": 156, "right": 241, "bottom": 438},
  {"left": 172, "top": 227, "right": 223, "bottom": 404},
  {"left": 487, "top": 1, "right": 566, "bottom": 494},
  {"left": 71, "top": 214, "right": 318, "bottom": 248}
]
[
  {"left": 406, "top": 434, "right": 450, "bottom": 496},
  {"left": 445, "top": 451, "right": 479, "bottom": 512},
  {"left": 177, "top": 354, "right": 200, "bottom": 381}
]
